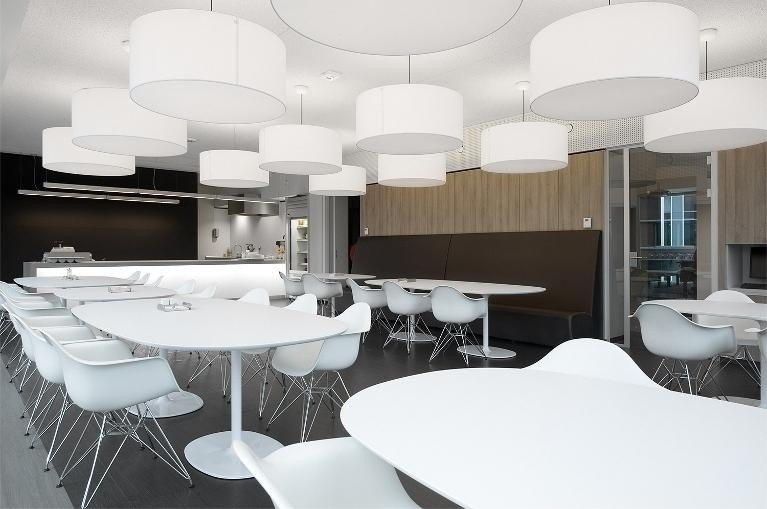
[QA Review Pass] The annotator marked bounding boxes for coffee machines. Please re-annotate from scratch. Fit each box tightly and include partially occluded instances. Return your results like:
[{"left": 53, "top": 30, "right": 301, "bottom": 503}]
[{"left": 275, "top": 240, "right": 285, "bottom": 259}]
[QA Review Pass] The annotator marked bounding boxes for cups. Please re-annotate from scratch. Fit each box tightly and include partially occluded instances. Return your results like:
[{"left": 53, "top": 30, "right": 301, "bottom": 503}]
[{"left": 241, "top": 250, "right": 264, "bottom": 259}]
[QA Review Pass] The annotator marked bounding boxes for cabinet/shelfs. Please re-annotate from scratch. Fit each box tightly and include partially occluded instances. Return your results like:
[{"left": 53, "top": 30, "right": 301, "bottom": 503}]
[{"left": 296, "top": 224, "right": 307, "bottom": 270}]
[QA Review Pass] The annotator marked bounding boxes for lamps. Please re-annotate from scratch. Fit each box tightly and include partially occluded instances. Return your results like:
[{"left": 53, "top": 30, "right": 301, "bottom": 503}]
[
  {"left": 352, "top": 55, "right": 463, "bottom": 156},
  {"left": 479, "top": 82, "right": 569, "bottom": 175},
  {"left": 71, "top": 89, "right": 187, "bottom": 157},
  {"left": 259, "top": 84, "right": 343, "bottom": 175},
  {"left": 17, "top": 154, "right": 180, "bottom": 204},
  {"left": 43, "top": 167, "right": 279, "bottom": 204},
  {"left": 309, "top": 164, "right": 365, "bottom": 197},
  {"left": 272, "top": 0, "right": 523, "bottom": 55},
  {"left": 42, "top": 126, "right": 135, "bottom": 177},
  {"left": 528, "top": 0, "right": 701, "bottom": 123},
  {"left": 128, "top": 0, "right": 286, "bottom": 124},
  {"left": 377, "top": 150, "right": 447, "bottom": 189},
  {"left": 643, "top": 30, "right": 767, "bottom": 153},
  {"left": 200, "top": 125, "right": 270, "bottom": 189}
]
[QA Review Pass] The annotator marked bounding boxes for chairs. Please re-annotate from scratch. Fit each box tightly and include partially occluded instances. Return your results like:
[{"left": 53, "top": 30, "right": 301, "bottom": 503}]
[
  {"left": 697, "top": 289, "right": 761, "bottom": 392},
  {"left": 628, "top": 303, "right": 735, "bottom": 396},
  {"left": 233, "top": 433, "right": 437, "bottom": 509},
  {"left": 679, "top": 250, "right": 697, "bottom": 297},
  {"left": 278, "top": 269, "right": 548, "bottom": 365}
]
